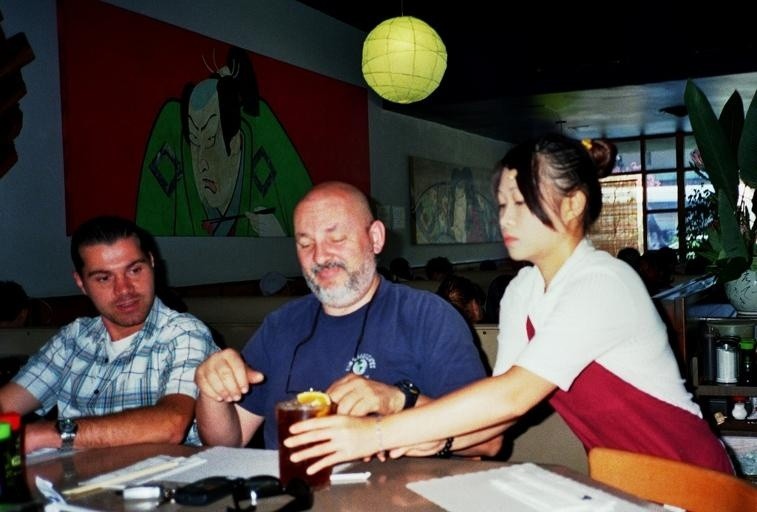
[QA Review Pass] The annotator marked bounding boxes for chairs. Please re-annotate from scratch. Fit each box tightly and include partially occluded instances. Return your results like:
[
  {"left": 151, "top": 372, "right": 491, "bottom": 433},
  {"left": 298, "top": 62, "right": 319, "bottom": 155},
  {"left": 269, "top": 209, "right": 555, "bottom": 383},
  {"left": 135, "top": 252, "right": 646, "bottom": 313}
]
[
  {"left": 466, "top": 322, "right": 590, "bottom": 469},
  {"left": 587, "top": 445, "right": 757, "bottom": 511}
]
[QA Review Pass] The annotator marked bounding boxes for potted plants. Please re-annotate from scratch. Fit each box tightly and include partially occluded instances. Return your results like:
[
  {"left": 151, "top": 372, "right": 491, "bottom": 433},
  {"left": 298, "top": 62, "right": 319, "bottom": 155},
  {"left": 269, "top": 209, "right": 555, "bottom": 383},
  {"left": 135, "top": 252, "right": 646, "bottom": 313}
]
[{"left": 683, "top": 78, "right": 757, "bottom": 318}]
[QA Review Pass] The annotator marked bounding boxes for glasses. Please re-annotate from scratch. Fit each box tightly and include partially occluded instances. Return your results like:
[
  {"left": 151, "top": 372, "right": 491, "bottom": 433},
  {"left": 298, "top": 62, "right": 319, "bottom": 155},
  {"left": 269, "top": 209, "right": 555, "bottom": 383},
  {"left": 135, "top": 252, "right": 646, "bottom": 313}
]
[{"left": 227, "top": 474, "right": 315, "bottom": 510}]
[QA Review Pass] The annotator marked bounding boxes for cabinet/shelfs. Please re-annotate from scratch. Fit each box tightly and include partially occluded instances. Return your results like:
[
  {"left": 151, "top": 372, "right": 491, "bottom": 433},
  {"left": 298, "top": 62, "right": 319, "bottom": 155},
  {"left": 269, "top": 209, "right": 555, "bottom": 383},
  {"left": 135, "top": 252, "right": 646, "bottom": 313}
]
[{"left": 666, "top": 296, "right": 757, "bottom": 478}]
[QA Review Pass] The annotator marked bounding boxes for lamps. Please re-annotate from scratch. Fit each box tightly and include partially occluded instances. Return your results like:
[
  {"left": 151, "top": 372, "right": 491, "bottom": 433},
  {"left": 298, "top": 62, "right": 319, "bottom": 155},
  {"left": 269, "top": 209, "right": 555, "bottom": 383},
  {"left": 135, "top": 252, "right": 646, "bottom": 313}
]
[{"left": 361, "top": 0, "right": 450, "bottom": 103}]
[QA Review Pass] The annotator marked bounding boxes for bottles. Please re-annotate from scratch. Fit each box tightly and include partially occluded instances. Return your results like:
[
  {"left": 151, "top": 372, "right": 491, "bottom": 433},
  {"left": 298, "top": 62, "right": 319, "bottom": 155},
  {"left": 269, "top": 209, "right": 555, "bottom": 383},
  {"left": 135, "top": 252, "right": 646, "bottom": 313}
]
[
  {"left": 0, "top": 410, "right": 24, "bottom": 489},
  {"left": 732, "top": 403, "right": 748, "bottom": 421},
  {"left": 698, "top": 325, "right": 757, "bottom": 385}
]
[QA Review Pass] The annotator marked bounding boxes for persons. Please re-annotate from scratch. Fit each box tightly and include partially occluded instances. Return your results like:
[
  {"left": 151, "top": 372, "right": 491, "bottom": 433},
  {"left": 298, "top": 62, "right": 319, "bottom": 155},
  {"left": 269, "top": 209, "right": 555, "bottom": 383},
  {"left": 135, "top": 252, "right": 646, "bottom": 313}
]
[
  {"left": 282, "top": 131, "right": 736, "bottom": 477},
  {"left": 389, "top": 257, "right": 412, "bottom": 281},
  {"left": 480, "top": 260, "right": 496, "bottom": 272},
  {"left": 617, "top": 247, "right": 640, "bottom": 266},
  {"left": 0, "top": 280, "right": 31, "bottom": 328},
  {"left": 0, "top": 214, "right": 222, "bottom": 455},
  {"left": 258, "top": 271, "right": 295, "bottom": 297},
  {"left": 136, "top": 48, "right": 314, "bottom": 237},
  {"left": 424, "top": 256, "right": 484, "bottom": 327},
  {"left": 195, "top": 179, "right": 503, "bottom": 458}
]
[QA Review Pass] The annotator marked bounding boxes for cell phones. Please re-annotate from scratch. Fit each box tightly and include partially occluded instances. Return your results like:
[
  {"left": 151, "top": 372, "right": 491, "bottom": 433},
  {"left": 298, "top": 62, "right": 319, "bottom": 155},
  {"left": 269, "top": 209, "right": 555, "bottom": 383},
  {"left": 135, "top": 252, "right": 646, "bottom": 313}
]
[{"left": 173, "top": 477, "right": 233, "bottom": 505}]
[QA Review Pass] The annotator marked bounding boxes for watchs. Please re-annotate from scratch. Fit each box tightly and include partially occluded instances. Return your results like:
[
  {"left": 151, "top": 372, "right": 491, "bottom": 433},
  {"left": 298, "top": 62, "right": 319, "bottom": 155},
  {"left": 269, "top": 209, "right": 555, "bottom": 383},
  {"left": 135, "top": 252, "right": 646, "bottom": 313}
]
[
  {"left": 55, "top": 417, "right": 78, "bottom": 452},
  {"left": 56, "top": 456, "right": 80, "bottom": 485},
  {"left": 393, "top": 379, "right": 421, "bottom": 411}
]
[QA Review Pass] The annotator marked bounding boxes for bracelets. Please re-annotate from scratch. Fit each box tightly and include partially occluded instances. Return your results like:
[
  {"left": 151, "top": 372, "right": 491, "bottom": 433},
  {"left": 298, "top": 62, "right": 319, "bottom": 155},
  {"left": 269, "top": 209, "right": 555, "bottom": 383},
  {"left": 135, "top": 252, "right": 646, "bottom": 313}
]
[{"left": 436, "top": 437, "right": 454, "bottom": 457}]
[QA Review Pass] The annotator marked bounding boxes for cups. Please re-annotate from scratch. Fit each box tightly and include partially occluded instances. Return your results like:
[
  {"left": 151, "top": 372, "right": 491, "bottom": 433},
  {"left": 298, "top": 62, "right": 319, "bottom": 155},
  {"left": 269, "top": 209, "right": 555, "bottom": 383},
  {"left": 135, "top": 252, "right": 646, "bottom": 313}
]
[{"left": 272, "top": 398, "right": 340, "bottom": 491}]
